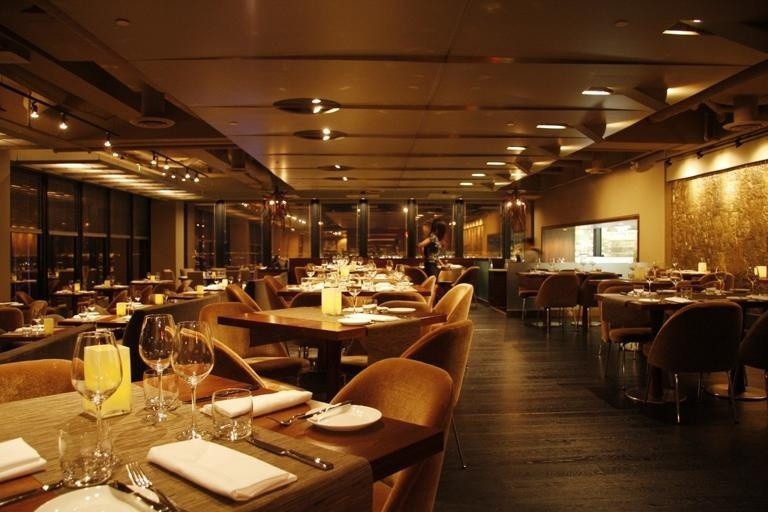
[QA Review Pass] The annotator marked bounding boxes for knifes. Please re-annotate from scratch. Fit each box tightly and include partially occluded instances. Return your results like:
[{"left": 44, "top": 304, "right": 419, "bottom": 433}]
[{"left": 246, "top": 435, "right": 334, "bottom": 472}]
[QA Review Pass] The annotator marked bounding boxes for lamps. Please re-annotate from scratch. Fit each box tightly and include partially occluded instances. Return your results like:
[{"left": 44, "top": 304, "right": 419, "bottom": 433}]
[
  {"left": 0, "top": 83, "right": 120, "bottom": 147},
  {"left": 114, "top": 151, "right": 189, "bottom": 182},
  {"left": 655, "top": 131, "right": 767, "bottom": 167},
  {"left": 148, "top": 150, "right": 208, "bottom": 182}
]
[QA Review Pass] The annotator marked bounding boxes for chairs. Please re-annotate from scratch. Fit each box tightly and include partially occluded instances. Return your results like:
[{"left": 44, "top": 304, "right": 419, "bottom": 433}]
[
  {"left": 517, "top": 269, "right": 768, "bottom": 426},
  {"left": 396, "top": 319, "right": 478, "bottom": 469},
  {"left": 1, "top": 356, "right": 83, "bottom": 404},
  {"left": 286, "top": 288, "right": 355, "bottom": 360},
  {"left": 200, "top": 301, "right": 309, "bottom": 385},
  {"left": 2, "top": 259, "right": 479, "bottom": 332},
  {"left": 328, "top": 355, "right": 457, "bottom": 509}
]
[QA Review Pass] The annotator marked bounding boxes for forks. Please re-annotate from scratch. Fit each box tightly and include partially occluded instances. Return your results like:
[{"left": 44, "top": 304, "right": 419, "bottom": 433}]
[
  {"left": 266, "top": 401, "right": 353, "bottom": 426},
  {"left": 124, "top": 461, "right": 185, "bottom": 512}
]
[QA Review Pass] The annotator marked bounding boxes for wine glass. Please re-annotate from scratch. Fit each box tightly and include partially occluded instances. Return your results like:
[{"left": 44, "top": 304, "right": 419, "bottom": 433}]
[
  {"left": 669, "top": 268, "right": 684, "bottom": 298},
  {"left": 346, "top": 278, "right": 361, "bottom": 317},
  {"left": 68, "top": 313, "right": 215, "bottom": 469},
  {"left": 78, "top": 299, "right": 95, "bottom": 322},
  {"left": 305, "top": 255, "right": 405, "bottom": 290},
  {"left": 534, "top": 257, "right": 540, "bottom": 273},
  {"left": 713, "top": 265, "right": 726, "bottom": 295},
  {"left": 642, "top": 266, "right": 657, "bottom": 298},
  {"left": 549, "top": 258, "right": 555, "bottom": 274},
  {"left": 747, "top": 267, "right": 760, "bottom": 299}
]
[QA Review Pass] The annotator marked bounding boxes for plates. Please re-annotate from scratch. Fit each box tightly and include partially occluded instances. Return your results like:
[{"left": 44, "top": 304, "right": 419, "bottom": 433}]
[
  {"left": 338, "top": 317, "right": 371, "bottom": 325},
  {"left": 34, "top": 484, "right": 161, "bottom": 512},
  {"left": 305, "top": 406, "right": 382, "bottom": 432},
  {"left": 388, "top": 307, "right": 416, "bottom": 314}
]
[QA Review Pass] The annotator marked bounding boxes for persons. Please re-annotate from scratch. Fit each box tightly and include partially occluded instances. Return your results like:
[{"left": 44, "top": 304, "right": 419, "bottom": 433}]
[
  {"left": 394, "top": 239, "right": 402, "bottom": 271},
  {"left": 523, "top": 237, "right": 540, "bottom": 265},
  {"left": 417, "top": 218, "right": 447, "bottom": 278}
]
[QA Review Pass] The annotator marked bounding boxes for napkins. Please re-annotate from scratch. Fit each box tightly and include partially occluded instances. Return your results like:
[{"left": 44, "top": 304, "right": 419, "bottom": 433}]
[
  {"left": 199, "top": 387, "right": 313, "bottom": 420},
  {"left": 147, "top": 439, "right": 298, "bottom": 501},
  {"left": 2, "top": 437, "right": 47, "bottom": 482}
]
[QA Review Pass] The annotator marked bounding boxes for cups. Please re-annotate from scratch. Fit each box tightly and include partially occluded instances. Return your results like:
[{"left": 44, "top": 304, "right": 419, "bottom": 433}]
[
  {"left": 363, "top": 298, "right": 378, "bottom": 315},
  {"left": 210, "top": 389, "right": 252, "bottom": 443},
  {"left": 59, "top": 421, "right": 110, "bottom": 488},
  {"left": 680, "top": 287, "right": 692, "bottom": 301}
]
